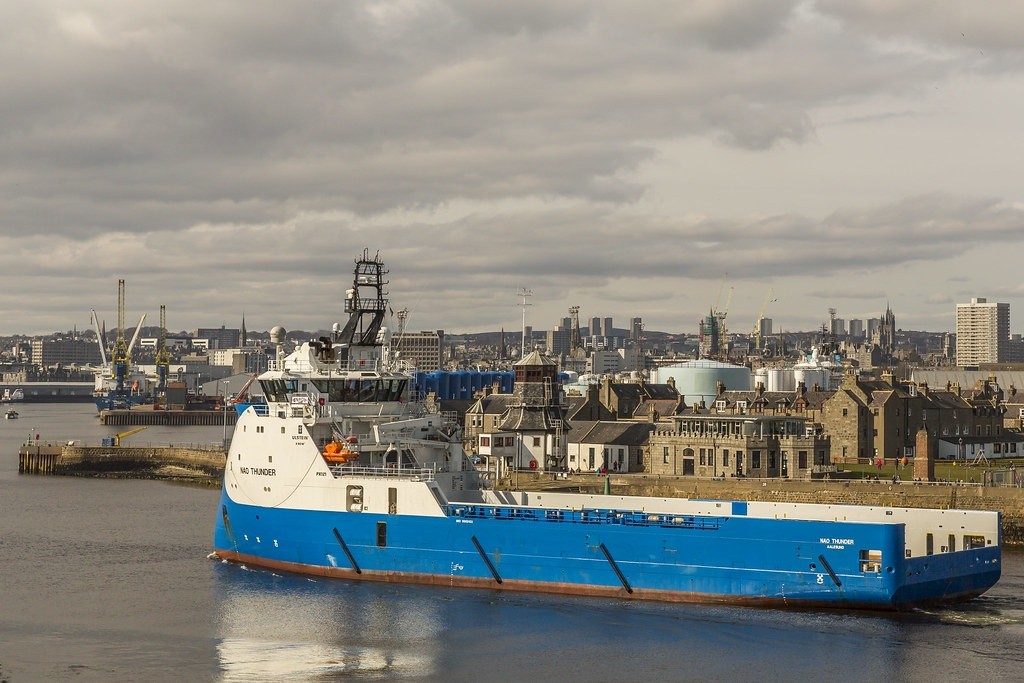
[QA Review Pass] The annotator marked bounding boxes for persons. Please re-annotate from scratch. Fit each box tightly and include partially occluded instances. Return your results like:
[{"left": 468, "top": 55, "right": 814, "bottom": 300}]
[
  {"left": 876, "top": 459, "right": 882, "bottom": 470},
  {"left": 719, "top": 471, "right": 725, "bottom": 481},
  {"left": 596, "top": 467, "right": 606, "bottom": 477},
  {"left": 894, "top": 457, "right": 898, "bottom": 470},
  {"left": 864, "top": 475, "right": 975, "bottom": 487},
  {"left": 613, "top": 460, "right": 621, "bottom": 472}
]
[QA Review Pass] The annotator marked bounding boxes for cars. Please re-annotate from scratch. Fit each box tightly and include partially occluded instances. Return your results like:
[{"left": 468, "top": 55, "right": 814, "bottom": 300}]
[
  {"left": 468, "top": 455, "right": 486, "bottom": 464},
  {"left": 188, "top": 386, "right": 203, "bottom": 395}
]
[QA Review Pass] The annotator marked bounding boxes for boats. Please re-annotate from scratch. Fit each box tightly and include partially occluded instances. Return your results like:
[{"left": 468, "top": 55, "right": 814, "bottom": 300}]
[
  {"left": 5, "top": 408, "right": 19, "bottom": 420},
  {"left": 1, "top": 388, "right": 33, "bottom": 403},
  {"left": 214, "top": 247, "right": 1001, "bottom": 613}
]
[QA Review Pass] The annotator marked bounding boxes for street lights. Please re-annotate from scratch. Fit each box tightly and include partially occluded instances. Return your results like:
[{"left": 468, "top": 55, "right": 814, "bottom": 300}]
[
  {"left": 516, "top": 433, "right": 520, "bottom": 490},
  {"left": 959, "top": 438, "right": 963, "bottom": 466},
  {"left": 517, "top": 287, "right": 533, "bottom": 355},
  {"left": 1007, "top": 367, "right": 1012, "bottom": 384},
  {"left": 223, "top": 381, "right": 229, "bottom": 450}
]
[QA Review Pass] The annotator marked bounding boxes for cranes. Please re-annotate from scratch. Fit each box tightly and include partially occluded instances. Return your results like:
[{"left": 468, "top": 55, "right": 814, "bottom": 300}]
[
  {"left": 716, "top": 287, "right": 734, "bottom": 346},
  {"left": 713, "top": 273, "right": 728, "bottom": 316},
  {"left": 751, "top": 288, "right": 777, "bottom": 349}
]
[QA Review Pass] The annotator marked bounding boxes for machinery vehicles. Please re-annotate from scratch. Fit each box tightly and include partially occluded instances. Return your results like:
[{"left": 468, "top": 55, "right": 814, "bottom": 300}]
[
  {"left": 155, "top": 305, "right": 170, "bottom": 378},
  {"left": 111, "top": 279, "right": 129, "bottom": 380},
  {"left": 90, "top": 308, "right": 112, "bottom": 376},
  {"left": 128, "top": 313, "right": 147, "bottom": 353}
]
